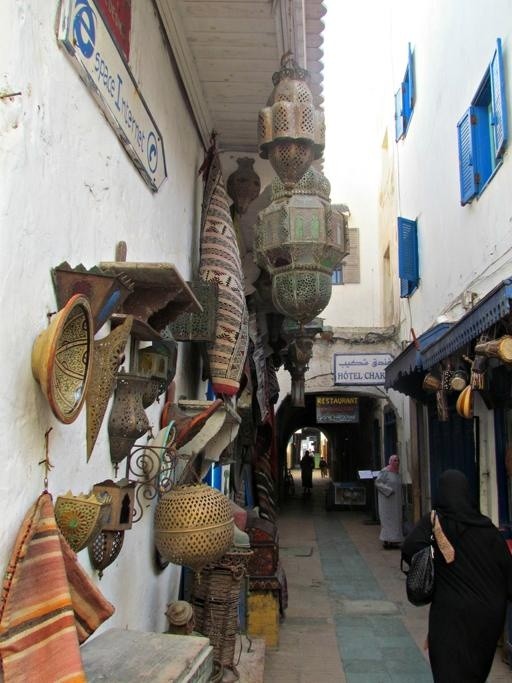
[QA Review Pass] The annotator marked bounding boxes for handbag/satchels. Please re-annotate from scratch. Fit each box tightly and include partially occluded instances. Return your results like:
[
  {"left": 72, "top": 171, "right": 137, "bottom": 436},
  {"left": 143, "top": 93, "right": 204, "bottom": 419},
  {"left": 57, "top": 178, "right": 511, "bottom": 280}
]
[{"left": 406, "top": 545, "right": 436, "bottom": 607}]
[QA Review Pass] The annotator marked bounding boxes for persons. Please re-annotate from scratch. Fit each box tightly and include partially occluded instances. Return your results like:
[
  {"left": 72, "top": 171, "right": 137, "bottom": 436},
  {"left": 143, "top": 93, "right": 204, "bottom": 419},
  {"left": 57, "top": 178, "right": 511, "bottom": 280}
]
[
  {"left": 300, "top": 450, "right": 315, "bottom": 494},
  {"left": 319, "top": 457, "right": 327, "bottom": 477},
  {"left": 398, "top": 466, "right": 511, "bottom": 681},
  {"left": 375, "top": 454, "right": 404, "bottom": 548}
]
